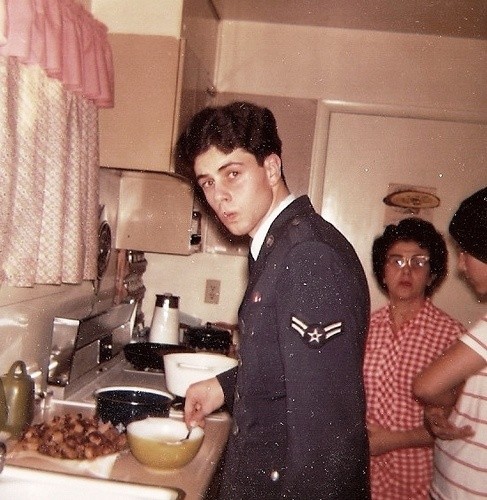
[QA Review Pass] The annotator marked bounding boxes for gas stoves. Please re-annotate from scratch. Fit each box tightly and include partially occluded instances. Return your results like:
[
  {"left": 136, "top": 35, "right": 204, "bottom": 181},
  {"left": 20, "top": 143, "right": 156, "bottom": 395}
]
[{"left": 46, "top": 293, "right": 237, "bottom": 421}]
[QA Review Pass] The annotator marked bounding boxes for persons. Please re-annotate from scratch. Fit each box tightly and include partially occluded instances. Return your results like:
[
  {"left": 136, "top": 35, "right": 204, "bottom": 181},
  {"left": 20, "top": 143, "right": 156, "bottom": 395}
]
[
  {"left": 175, "top": 101, "right": 371, "bottom": 500},
  {"left": 414, "top": 188, "right": 487, "bottom": 500},
  {"left": 362, "top": 216, "right": 469, "bottom": 500}
]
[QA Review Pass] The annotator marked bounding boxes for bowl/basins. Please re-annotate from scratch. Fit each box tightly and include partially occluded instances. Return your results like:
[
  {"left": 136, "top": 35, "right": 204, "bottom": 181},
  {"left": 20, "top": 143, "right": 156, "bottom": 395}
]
[
  {"left": 126, "top": 417, "right": 204, "bottom": 474},
  {"left": 93, "top": 386, "right": 174, "bottom": 426},
  {"left": 162, "top": 352, "right": 237, "bottom": 398}
]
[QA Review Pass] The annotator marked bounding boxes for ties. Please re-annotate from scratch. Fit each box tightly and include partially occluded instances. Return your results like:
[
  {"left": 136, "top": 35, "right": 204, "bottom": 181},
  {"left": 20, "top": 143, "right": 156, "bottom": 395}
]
[{"left": 246, "top": 247, "right": 255, "bottom": 272}]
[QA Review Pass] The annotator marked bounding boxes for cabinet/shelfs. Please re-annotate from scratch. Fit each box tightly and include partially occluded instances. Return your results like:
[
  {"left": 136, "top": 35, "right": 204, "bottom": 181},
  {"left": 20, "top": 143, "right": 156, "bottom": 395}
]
[{"left": 90, "top": 0, "right": 320, "bottom": 258}]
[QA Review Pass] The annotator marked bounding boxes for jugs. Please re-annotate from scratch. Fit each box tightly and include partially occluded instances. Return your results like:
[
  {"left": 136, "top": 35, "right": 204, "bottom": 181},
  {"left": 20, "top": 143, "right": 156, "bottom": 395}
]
[
  {"left": 0, "top": 360, "right": 36, "bottom": 434},
  {"left": 148, "top": 293, "right": 180, "bottom": 345}
]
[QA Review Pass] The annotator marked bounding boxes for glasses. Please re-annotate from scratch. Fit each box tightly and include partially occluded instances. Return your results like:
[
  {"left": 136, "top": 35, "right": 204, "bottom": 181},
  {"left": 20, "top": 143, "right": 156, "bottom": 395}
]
[{"left": 386, "top": 254, "right": 431, "bottom": 271}]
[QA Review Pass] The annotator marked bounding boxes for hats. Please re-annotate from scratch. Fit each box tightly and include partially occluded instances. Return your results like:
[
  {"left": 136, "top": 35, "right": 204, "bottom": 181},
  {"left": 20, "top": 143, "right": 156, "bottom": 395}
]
[{"left": 449, "top": 187, "right": 487, "bottom": 263}]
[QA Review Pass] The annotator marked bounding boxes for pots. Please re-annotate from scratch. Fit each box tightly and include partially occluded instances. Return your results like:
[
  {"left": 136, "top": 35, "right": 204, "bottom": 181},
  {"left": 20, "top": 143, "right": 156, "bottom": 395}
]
[
  {"left": 122, "top": 343, "right": 195, "bottom": 370},
  {"left": 184, "top": 320, "right": 231, "bottom": 354}
]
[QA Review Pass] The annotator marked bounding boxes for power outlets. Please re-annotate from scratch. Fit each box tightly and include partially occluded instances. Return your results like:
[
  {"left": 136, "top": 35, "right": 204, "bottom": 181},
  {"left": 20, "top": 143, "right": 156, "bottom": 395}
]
[{"left": 205, "top": 279, "right": 221, "bottom": 303}]
